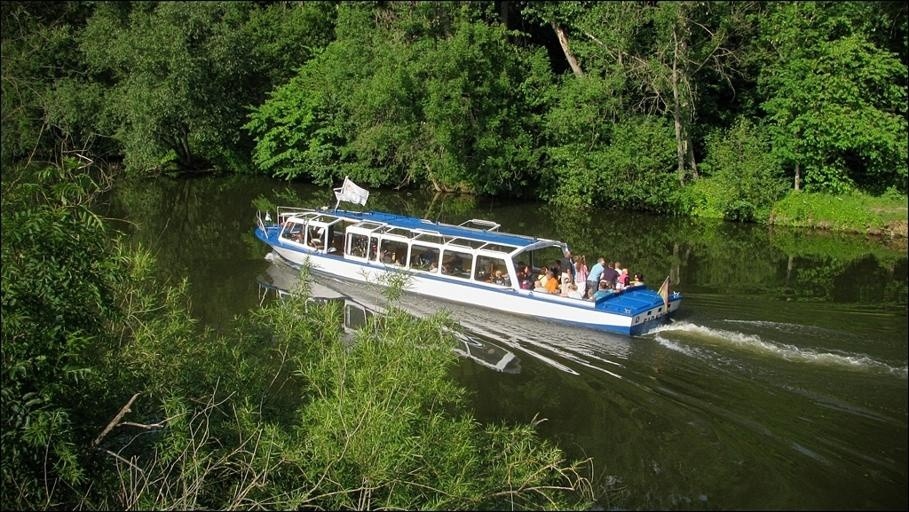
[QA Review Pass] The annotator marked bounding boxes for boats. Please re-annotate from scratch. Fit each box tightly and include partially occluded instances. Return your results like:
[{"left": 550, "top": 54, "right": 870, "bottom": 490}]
[{"left": 255, "top": 174, "right": 683, "bottom": 337}]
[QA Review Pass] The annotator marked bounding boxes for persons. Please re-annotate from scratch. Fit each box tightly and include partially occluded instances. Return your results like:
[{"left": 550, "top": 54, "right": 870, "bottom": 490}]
[{"left": 494, "top": 251, "right": 644, "bottom": 302}]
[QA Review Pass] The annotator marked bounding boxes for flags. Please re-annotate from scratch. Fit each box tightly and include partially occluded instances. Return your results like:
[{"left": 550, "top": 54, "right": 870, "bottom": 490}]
[
  {"left": 339, "top": 179, "right": 369, "bottom": 206},
  {"left": 659, "top": 278, "right": 670, "bottom": 313}
]
